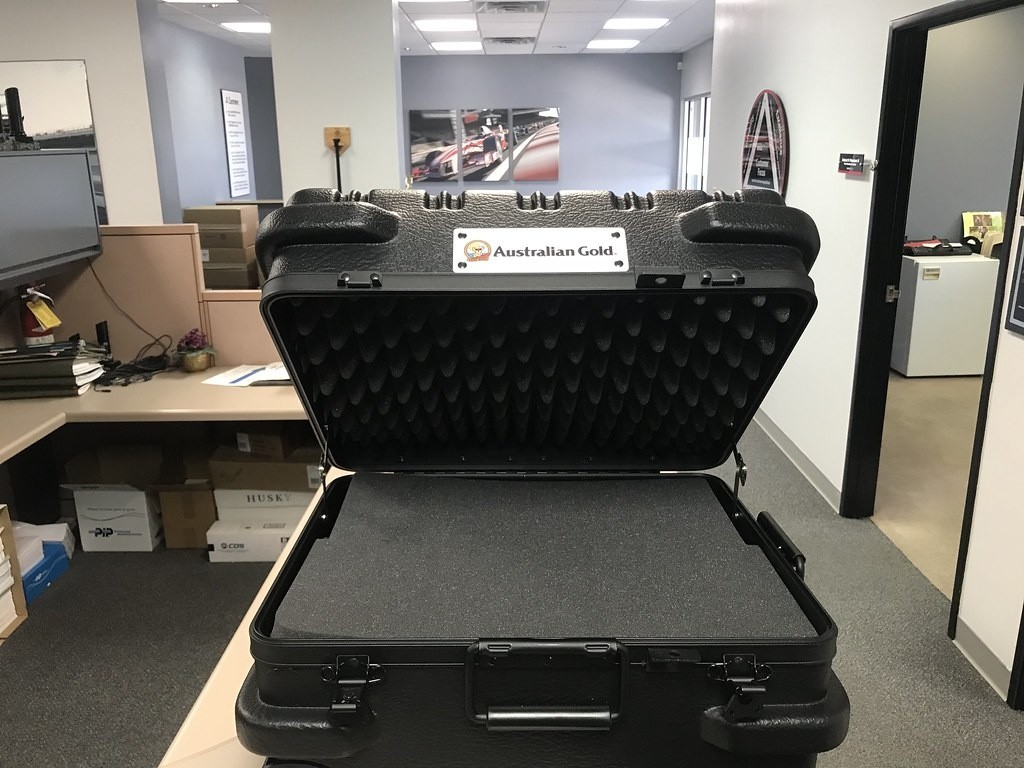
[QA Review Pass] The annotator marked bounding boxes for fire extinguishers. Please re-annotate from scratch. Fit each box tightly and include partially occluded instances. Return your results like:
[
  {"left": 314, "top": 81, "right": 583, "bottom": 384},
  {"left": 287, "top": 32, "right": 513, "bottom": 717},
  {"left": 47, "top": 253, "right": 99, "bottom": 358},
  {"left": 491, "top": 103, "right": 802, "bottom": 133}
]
[{"left": 0, "top": 283, "right": 56, "bottom": 346}]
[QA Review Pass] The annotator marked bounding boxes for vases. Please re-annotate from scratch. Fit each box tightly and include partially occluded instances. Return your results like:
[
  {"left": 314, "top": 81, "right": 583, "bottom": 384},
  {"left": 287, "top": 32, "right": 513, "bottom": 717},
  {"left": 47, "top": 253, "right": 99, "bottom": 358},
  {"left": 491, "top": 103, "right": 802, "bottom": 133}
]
[{"left": 181, "top": 353, "right": 211, "bottom": 372}]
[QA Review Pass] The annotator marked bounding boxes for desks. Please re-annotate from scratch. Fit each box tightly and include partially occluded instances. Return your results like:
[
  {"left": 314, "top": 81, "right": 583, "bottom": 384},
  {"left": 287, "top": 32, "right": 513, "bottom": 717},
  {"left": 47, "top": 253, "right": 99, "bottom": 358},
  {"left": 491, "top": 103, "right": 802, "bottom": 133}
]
[
  {"left": 157, "top": 466, "right": 678, "bottom": 768},
  {"left": 0, "top": 367, "right": 308, "bottom": 465}
]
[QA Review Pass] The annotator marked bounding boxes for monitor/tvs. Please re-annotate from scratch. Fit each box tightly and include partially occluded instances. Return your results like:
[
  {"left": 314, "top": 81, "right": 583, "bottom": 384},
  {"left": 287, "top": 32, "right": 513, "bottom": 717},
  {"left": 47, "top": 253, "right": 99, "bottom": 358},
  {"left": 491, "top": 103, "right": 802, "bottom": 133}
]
[{"left": 0, "top": 149, "right": 103, "bottom": 292}]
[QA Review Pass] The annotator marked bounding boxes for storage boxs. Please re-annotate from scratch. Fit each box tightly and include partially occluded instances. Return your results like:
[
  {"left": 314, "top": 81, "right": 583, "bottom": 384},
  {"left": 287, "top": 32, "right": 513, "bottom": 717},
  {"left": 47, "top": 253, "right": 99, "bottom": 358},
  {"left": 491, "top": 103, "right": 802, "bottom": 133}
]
[
  {"left": 201, "top": 244, "right": 259, "bottom": 287},
  {"left": 0, "top": 504, "right": 29, "bottom": 647},
  {"left": 183, "top": 205, "right": 260, "bottom": 247},
  {"left": 205, "top": 521, "right": 300, "bottom": 563},
  {"left": 236, "top": 422, "right": 309, "bottom": 459},
  {"left": 36, "top": 523, "right": 76, "bottom": 560},
  {"left": 59, "top": 482, "right": 164, "bottom": 553},
  {"left": 208, "top": 430, "right": 324, "bottom": 493},
  {"left": 180, "top": 442, "right": 214, "bottom": 482},
  {"left": 21, "top": 544, "right": 70, "bottom": 604},
  {"left": 162, "top": 484, "right": 216, "bottom": 549},
  {"left": 213, "top": 488, "right": 321, "bottom": 524}
]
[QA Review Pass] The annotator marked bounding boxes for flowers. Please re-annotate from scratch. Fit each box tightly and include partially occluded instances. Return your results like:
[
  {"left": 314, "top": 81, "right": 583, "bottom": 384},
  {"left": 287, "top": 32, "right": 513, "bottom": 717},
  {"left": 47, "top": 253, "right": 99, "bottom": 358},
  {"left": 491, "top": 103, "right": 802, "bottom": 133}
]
[{"left": 169, "top": 327, "right": 219, "bottom": 359}]
